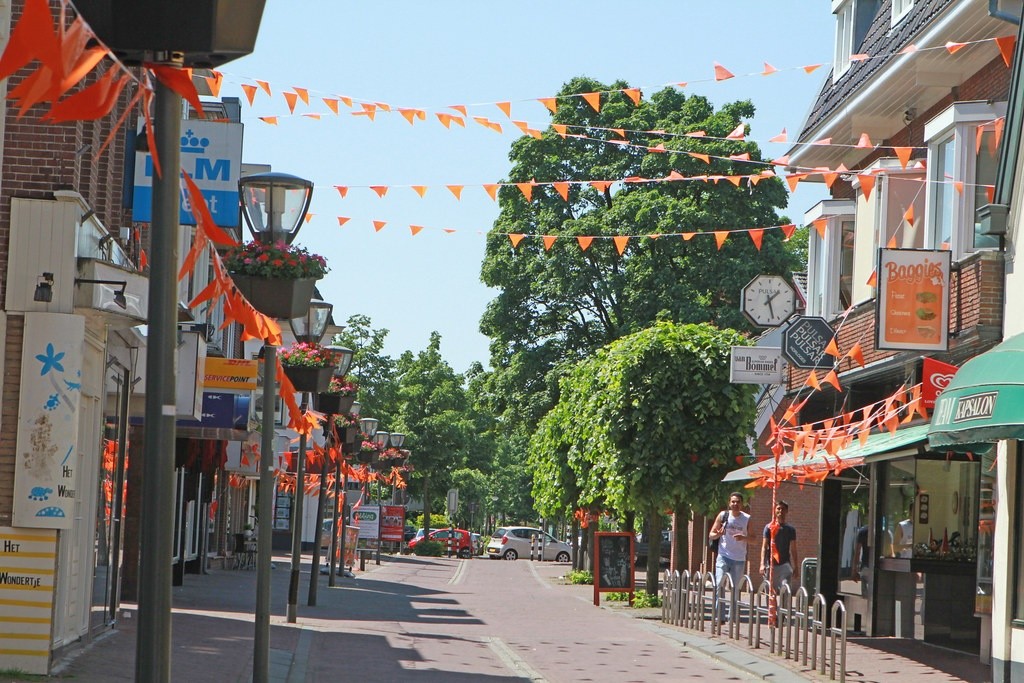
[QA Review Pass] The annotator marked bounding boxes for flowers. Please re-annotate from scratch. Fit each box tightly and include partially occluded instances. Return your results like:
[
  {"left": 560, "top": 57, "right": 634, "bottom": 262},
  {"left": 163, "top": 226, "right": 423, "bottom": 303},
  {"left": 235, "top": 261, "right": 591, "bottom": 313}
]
[
  {"left": 276, "top": 341, "right": 343, "bottom": 367},
  {"left": 349, "top": 441, "right": 385, "bottom": 455},
  {"left": 355, "top": 430, "right": 369, "bottom": 441},
  {"left": 319, "top": 411, "right": 363, "bottom": 429},
  {"left": 378, "top": 448, "right": 413, "bottom": 474},
  {"left": 221, "top": 238, "right": 333, "bottom": 280},
  {"left": 322, "top": 375, "right": 358, "bottom": 401}
]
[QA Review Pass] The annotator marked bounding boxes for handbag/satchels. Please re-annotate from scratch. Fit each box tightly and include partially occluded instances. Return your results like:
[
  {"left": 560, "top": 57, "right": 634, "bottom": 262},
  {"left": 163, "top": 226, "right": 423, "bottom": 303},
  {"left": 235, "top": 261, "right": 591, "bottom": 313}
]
[{"left": 709, "top": 509, "right": 729, "bottom": 552}]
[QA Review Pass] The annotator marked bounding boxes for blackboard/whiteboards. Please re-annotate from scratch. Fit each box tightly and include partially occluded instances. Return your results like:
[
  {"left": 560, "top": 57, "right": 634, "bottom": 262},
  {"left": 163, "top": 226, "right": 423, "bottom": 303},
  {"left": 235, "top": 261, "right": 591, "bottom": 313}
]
[{"left": 593, "top": 532, "right": 635, "bottom": 592}]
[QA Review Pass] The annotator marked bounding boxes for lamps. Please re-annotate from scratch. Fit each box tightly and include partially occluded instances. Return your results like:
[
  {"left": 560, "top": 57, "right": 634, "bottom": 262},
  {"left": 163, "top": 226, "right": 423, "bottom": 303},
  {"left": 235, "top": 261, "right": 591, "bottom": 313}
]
[
  {"left": 75, "top": 278, "right": 127, "bottom": 310},
  {"left": 33, "top": 271, "right": 54, "bottom": 302},
  {"left": 120, "top": 227, "right": 130, "bottom": 246}
]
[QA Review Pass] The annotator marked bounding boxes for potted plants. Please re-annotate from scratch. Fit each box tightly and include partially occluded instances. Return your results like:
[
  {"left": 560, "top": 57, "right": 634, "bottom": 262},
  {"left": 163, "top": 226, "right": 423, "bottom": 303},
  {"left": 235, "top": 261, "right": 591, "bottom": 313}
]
[{"left": 244, "top": 524, "right": 252, "bottom": 536}]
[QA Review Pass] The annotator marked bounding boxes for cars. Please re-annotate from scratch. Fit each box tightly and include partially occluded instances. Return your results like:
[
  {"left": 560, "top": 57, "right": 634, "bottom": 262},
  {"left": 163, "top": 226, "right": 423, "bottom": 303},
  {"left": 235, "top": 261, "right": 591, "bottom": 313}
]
[
  {"left": 319, "top": 519, "right": 332, "bottom": 548},
  {"left": 408, "top": 529, "right": 473, "bottom": 558},
  {"left": 472, "top": 534, "right": 484, "bottom": 556},
  {"left": 486, "top": 525, "right": 572, "bottom": 562}
]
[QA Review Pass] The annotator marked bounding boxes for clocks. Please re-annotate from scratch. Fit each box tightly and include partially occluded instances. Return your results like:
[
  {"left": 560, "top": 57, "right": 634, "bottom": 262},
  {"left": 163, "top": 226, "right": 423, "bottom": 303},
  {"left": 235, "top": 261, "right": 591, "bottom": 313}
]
[{"left": 740, "top": 274, "right": 796, "bottom": 328}]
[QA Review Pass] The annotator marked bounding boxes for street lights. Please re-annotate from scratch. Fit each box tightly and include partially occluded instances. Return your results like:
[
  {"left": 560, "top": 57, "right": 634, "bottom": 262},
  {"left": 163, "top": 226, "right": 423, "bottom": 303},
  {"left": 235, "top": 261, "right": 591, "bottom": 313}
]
[
  {"left": 308, "top": 345, "right": 354, "bottom": 606},
  {"left": 373, "top": 432, "right": 407, "bottom": 566},
  {"left": 287, "top": 298, "right": 334, "bottom": 625},
  {"left": 73, "top": 0, "right": 264, "bottom": 683},
  {"left": 233, "top": 169, "right": 315, "bottom": 682},
  {"left": 329, "top": 401, "right": 377, "bottom": 586}
]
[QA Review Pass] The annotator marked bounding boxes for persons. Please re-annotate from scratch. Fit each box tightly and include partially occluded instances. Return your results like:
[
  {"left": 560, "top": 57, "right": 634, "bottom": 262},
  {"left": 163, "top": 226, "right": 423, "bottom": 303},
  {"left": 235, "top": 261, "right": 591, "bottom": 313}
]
[
  {"left": 710, "top": 492, "right": 758, "bottom": 629},
  {"left": 852, "top": 502, "right": 916, "bottom": 594},
  {"left": 760, "top": 502, "right": 799, "bottom": 627}
]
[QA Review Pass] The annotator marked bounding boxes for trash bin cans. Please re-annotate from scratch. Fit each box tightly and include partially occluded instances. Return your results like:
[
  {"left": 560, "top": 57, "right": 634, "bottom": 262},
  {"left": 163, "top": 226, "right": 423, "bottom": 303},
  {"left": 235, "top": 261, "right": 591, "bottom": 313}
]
[{"left": 801, "top": 558, "right": 817, "bottom": 606}]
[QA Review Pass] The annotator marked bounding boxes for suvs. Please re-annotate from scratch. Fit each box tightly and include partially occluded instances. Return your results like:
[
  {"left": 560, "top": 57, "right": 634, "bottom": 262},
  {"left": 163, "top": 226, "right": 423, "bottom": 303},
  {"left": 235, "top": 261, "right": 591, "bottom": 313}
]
[
  {"left": 397, "top": 526, "right": 416, "bottom": 548},
  {"left": 417, "top": 528, "right": 436, "bottom": 536}
]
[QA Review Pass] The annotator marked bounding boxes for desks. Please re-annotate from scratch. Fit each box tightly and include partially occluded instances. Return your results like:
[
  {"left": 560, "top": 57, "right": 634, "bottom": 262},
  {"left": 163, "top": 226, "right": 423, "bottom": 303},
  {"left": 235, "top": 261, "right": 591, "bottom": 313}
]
[{"left": 237, "top": 540, "right": 257, "bottom": 569}]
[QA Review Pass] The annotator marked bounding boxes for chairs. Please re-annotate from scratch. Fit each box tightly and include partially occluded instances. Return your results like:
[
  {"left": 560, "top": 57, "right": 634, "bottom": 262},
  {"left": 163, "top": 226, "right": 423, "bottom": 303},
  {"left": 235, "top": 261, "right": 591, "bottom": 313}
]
[
  {"left": 523, "top": 531, "right": 530, "bottom": 538},
  {"left": 516, "top": 530, "right": 523, "bottom": 537},
  {"left": 232, "top": 533, "right": 257, "bottom": 570}
]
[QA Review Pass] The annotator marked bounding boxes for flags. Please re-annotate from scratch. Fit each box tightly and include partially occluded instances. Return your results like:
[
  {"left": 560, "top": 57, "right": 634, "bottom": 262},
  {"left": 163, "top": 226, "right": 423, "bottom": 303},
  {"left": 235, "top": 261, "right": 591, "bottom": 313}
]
[{"left": 921, "top": 356, "right": 959, "bottom": 407}]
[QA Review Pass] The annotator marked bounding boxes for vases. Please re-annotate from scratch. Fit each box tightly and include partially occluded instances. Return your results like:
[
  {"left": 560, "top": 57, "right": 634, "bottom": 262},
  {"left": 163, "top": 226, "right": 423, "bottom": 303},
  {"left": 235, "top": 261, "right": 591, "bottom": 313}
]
[
  {"left": 317, "top": 394, "right": 356, "bottom": 415},
  {"left": 399, "top": 471, "right": 409, "bottom": 478},
  {"left": 341, "top": 438, "right": 363, "bottom": 453},
  {"left": 371, "top": 459, "right": 393, "bottom": 469},
  {"left": 357, "top": 450, "right": 382, "bottom": 462},
  {"left": 230, "top": 272, "right": 320, "bottom": 321},
  {"left": 323, "top": 426, "right": 360, "bottom": 443},
  {"left": 283, "top": 365, "right": 338, "bottom": 392},
  {"left": 391, "top": 458, "right": 407, "bottom": 467}
]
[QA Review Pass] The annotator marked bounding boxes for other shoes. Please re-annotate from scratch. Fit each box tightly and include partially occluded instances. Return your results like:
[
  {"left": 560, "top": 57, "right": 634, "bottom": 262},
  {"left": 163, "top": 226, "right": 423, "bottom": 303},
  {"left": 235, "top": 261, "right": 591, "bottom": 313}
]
[{"left": 711, "top": 619, "right": 725, "bottom": 626}]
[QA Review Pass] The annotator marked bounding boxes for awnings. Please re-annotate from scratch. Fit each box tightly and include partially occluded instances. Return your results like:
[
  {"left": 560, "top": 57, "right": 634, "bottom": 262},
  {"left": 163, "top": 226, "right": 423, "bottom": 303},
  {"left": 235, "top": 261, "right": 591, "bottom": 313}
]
[
  {"left": 926, "top": 332, "right": 1024, "bottom": 454},
  {"left": 719, "top": 424, "right": 930, "bottom": 482}
]
